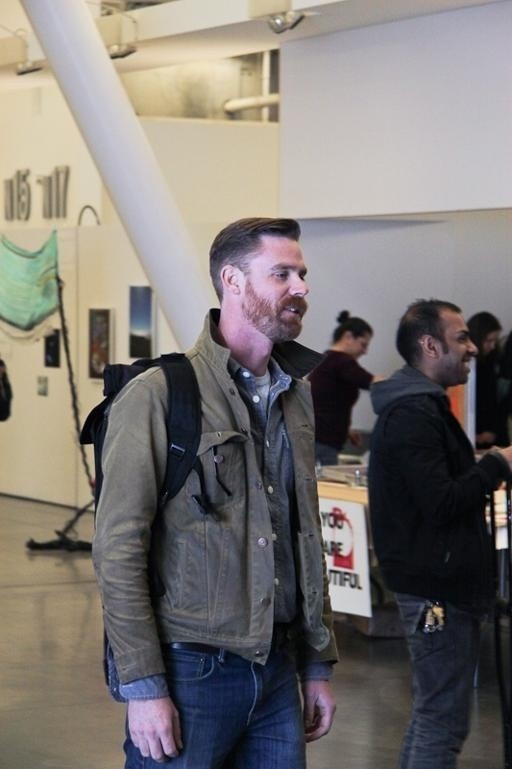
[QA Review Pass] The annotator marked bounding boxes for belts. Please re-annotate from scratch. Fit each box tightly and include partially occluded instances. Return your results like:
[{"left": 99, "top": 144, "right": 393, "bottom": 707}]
[{"left": 166, "top": 642, "right": 235, "bottom": 659}]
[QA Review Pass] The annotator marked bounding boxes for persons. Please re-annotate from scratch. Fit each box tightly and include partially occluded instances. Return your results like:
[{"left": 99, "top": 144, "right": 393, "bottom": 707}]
[
  {"left": 366, "top": 299, "right": 512, "bottom": 768},
  {"left": 466, "top": 311, "right": 512, "bottom": 449},
  {"left": 307, "top": 311, "right": 388, "bottom": 466},
  {"left": 91, "top": 214, "right": 344, "bottom": 768}
]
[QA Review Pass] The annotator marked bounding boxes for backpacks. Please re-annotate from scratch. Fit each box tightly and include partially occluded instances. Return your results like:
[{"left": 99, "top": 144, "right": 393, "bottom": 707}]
[{"left": 80, "top": 353, "right": 212, "bottom": 526}]
